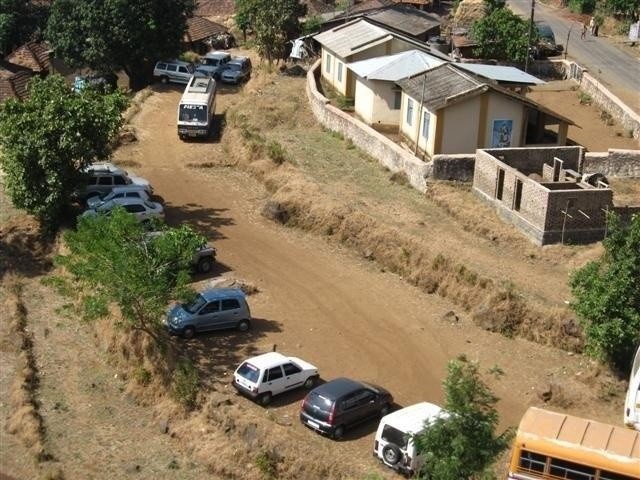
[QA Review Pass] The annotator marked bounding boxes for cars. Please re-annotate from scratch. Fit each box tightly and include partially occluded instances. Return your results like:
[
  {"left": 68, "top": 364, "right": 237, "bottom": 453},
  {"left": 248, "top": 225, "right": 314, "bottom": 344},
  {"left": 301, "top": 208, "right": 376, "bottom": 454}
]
[
  {"left": 68, "top": 162, "right": 217, "bottom": 288},
  {"left": 232, "top": 351, "right": 394, "bottom": 440},
  {"left": 152, "top": 50, "right": 252, "bottom": 85},
  {"left": 164, "top": 287, "right": 252, "bottom": 339}
]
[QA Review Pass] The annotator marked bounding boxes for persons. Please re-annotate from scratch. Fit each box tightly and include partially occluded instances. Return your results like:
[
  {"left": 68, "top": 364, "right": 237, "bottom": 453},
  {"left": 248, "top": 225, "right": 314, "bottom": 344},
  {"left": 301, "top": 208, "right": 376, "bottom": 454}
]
[{"left": 581, "top": 17, "right": 599, "bottom": 39}]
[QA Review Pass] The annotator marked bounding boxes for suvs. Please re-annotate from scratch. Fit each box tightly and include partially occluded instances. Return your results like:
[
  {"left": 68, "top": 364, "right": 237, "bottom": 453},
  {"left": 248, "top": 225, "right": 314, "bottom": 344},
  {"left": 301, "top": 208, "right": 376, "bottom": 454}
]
[{"left": 375, "top": 401, "right": 464, "bottom": 478}]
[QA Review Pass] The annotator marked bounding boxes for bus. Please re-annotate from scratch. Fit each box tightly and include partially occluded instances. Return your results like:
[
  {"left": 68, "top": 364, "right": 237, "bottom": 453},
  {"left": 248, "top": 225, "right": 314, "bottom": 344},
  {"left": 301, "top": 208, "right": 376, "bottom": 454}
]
[
  {"left": 507, "top": 346, "right": 640, "bottom": 480},
  {"left": 177, "top": 73, "right": 217, "bottom": 141}
]
